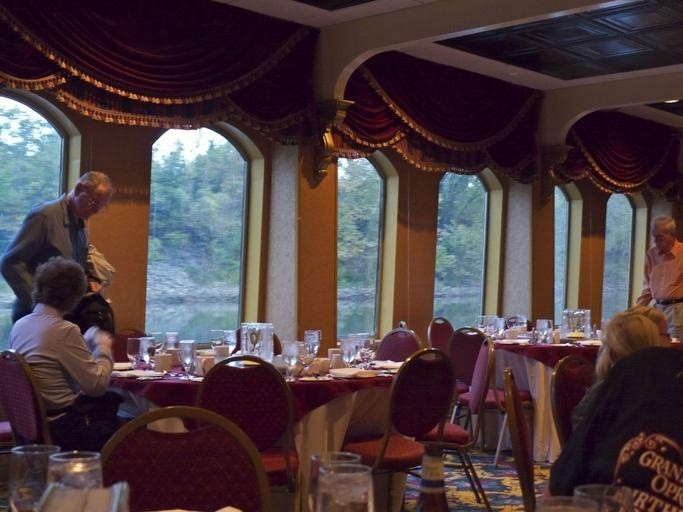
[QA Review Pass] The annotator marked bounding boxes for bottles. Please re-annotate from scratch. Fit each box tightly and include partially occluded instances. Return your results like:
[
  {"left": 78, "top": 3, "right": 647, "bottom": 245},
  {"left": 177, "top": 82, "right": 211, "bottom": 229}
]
[{"left": 411, "top": 444, "right": 452, "bottom": 510}]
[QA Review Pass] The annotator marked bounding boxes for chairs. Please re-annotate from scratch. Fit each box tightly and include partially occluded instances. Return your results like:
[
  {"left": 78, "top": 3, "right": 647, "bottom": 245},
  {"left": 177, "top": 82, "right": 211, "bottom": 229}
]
[{"left": 0, "top": 322, "right": 683, "bottom": 512}]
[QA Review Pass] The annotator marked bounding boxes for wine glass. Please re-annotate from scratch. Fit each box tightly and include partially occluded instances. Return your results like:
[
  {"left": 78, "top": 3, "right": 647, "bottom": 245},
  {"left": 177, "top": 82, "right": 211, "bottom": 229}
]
[
  {"left": 7, "top": 442, "right": 60, "bottom": 510},
  {"left": 47, "top": 448, "right": 104, "bottom": 493},
  {"left": 125, "top": 327, "right": 235, "bottom": 382},
  {"left": 280, "top": 329, "right": 376, "bottom": 382},
  {"left": 307, "top": 447, "right": 376, "bottom": 512}
]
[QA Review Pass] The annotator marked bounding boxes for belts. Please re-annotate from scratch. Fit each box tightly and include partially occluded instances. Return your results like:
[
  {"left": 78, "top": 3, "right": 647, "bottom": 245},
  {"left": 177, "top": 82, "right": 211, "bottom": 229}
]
[{"left": 656, "top": 298, "right": 683, "bottom": 305}]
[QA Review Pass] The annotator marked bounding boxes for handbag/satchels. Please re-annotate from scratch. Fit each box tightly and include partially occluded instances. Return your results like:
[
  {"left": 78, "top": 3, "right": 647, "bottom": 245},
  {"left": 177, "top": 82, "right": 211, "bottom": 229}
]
[{"left": 72, "top": 295, "right": 114, "bottom": 337}]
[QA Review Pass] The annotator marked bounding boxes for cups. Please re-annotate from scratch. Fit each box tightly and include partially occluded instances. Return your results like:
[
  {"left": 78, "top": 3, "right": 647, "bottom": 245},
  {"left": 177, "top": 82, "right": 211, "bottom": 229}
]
[
  {"left": 560, "top": 309, "right": 590, "bottom": 337},
  {"left": 241, "top": 322, "right": 274, "bottom": 363},
  {"left": 473, "top": 314, "right": 504, "bottom": 340},
  {"left": 535, "top": 319, "right": 554, "bottom": 342},
  {"left": 574, "top": 485, "right": 634, "bottom": 511},
  {"left": 552, "top": 330, "right": 560, "bottom": 343},
  {"left": 506, "top": 328, "right": 519, "bottom": 339}
]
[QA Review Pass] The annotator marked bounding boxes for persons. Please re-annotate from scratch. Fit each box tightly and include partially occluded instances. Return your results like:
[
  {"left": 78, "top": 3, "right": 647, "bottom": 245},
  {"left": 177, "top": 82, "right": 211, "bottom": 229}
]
[
  {"left": 0, "top": 171, "right": 114, "bottom": 338},
  {"left": 549, "top": 299, "right": 683, "bottom": 512},
  {"left": 7, "top": 257, "right": 125, "bottom": 451},
  {"left": 636, "top": 215, "right": 682, "bottom": 339}
]
